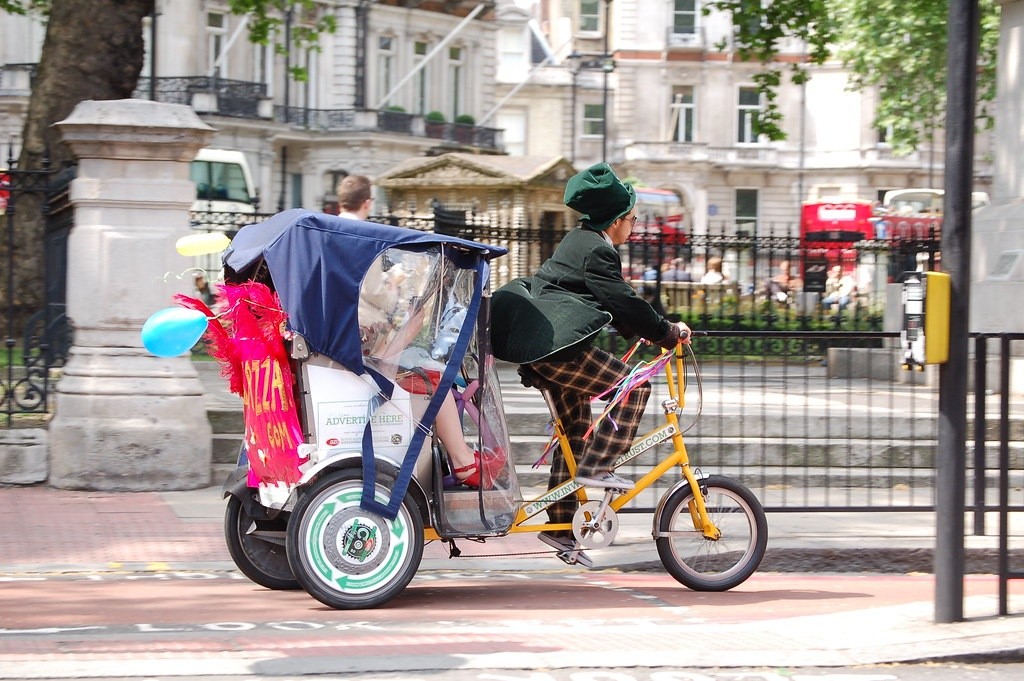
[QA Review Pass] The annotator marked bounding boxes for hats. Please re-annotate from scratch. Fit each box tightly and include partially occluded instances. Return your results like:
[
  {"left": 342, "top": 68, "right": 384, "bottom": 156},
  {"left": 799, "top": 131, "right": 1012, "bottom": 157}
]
[{"left": 562, "top": 163, "right": 635, "bottom": 232}]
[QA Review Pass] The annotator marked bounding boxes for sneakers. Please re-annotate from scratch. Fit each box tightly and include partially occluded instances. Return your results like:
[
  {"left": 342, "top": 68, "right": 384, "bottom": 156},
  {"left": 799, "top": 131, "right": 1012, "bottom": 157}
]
[
  {"left": 538, "top": 532, "right": 594, "bottom": 568},
  {"left": 575, "top": 471, "right": 635, "bottom": 488}
]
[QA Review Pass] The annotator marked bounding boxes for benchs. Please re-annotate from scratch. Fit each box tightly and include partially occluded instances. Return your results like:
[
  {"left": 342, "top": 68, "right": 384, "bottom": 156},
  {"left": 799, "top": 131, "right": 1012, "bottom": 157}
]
[
  {"left": 295, "top": 342, "right": 428, "bottom": 490},
  {"left": 630, "top": 277, "right": 739, "bottom": 311}
]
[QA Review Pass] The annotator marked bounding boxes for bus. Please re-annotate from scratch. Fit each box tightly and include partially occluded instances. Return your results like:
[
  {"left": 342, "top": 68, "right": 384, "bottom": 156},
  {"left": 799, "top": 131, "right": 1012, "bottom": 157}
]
[
  {"left": 192, "top": 150, "right": 261, "bottom": 280},
  {"left": 628, "top": 186, "right": 691, "bottom": 264},
  {"left": 802, "top": 201, "right": 942, "bottom": 294}
]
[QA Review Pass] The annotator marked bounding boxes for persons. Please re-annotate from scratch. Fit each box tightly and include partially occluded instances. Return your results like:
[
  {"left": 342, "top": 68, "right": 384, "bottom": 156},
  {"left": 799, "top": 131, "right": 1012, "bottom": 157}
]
[
  {"left": 821, "top": 265, "right": 857, "bottom": 308},
  {"left": 529, "top": 162, "right": 692, "bottom": 568},
  {"left": 191, "top": 273, "right": 219, "bottom": 309},
  {"left": 358, "top": 259, "right": 507, "bottom": 490},
  {"left": 621, "top": 255, "right": 725, "bottom": 284},
  {"left": 775, "top": 259, "right": 803, "bottom": 291},
  {"left": 336, "top": 176, "right": 372, "bottom": 221}
]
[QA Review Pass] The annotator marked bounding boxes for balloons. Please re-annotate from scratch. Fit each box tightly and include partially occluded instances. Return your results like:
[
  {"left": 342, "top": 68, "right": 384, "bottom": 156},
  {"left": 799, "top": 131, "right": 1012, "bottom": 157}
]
[
  {"left": 443, "top": 376, "right": 495, "bottom": 486},
  {"left": 175, "top": 233, "right": 231, "bottom": 257},
  {"left": 140, "top": 307, "right": 208, "bottom": 359}
]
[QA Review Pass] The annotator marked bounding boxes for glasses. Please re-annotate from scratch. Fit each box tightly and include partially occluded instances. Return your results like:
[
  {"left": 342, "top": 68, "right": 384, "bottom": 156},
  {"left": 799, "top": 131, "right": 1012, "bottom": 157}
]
[{"left": 625, "top": 216, "right": 637, "bottom": 226}]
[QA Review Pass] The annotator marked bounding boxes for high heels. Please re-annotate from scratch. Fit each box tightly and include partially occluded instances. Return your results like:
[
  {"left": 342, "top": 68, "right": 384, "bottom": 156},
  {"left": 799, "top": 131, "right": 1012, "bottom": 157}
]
[{"left": 454, "top": 445, "right": 506, "bottom": 490}]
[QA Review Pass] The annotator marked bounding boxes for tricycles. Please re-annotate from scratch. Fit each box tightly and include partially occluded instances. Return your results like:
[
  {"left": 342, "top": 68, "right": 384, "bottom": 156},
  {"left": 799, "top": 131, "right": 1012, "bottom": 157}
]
[{"left": 223, "top": 210, "right": 770, "bottom": 608}]
